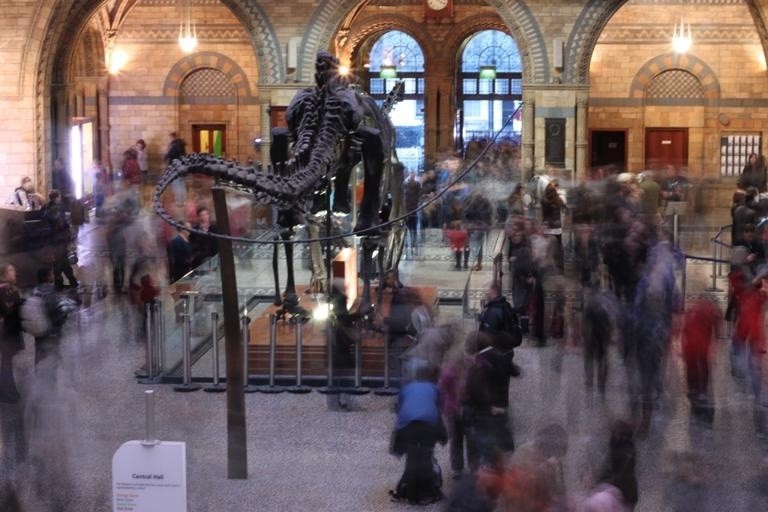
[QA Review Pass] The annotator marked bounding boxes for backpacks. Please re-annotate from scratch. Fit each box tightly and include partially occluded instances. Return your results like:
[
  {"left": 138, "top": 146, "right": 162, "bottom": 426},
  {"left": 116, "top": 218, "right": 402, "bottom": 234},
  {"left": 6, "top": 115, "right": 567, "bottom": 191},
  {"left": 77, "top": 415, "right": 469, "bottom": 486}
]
[{"left": 20, "top": 287, "right": 53, "bottom": 338}]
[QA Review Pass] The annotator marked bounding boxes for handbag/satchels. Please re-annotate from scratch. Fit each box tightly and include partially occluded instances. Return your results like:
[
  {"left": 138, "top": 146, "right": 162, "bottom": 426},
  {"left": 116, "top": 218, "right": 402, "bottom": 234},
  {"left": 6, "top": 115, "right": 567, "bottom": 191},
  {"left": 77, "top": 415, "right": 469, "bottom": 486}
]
[
  {"left": 392, "top": 453, "right": 442, "bottom": 496},
  {"left": 489, "top": 304, "right": 522, "bottom": 352}
]
